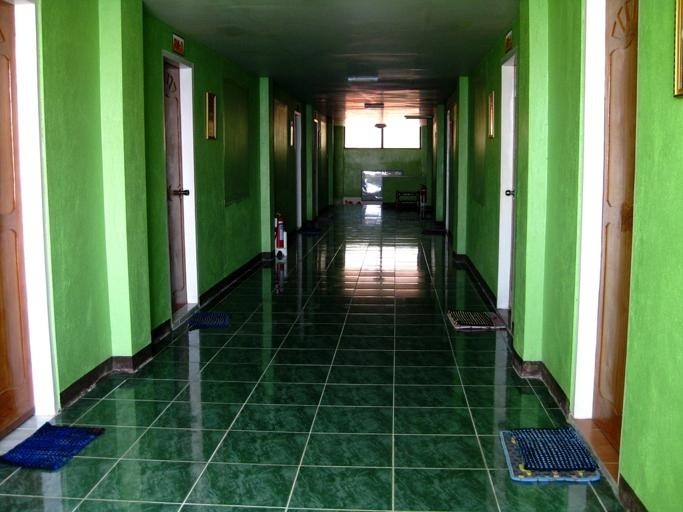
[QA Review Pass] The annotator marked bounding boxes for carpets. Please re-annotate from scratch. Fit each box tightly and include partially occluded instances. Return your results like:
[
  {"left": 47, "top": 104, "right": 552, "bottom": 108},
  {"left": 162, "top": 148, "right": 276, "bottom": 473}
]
[
  {"left": 185, "top": 307, "right": 233, "bottom": 330},
  {"left": 420, "top": 227, "right": 446, "bottom": 237},
  {"left": 444, "top": 308, "right": 506, "bottom": 334},
  {"left": 0, "top": 418, "right": 108, "bottom": 476},
  {"left": 496, "top": 423, "right": 600, "bottom": 486},
  {"left": 298, "top": 219, "right": 330, "bottom": 237}
]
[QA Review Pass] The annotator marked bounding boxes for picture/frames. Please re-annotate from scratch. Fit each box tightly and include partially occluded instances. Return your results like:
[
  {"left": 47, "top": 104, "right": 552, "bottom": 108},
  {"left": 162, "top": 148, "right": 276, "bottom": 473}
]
[
  {"left": 487, "top": 91, "right": 494, "bottom": 140},
  {"left": 205, "top": 92, "right": 217, "bottom": 142},
  {"left": 674, "top": 0, "right": 683, "bottom": 97}
]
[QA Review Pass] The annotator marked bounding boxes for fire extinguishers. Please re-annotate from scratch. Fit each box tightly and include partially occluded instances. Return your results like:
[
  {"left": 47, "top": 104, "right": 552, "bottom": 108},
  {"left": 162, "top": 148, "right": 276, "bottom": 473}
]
[
  {"left": 274, "top": 212, "right": 284, "bottom": 248},
  {"left": 417, "top": 183, "right": 427, "bottom": 202}
]
